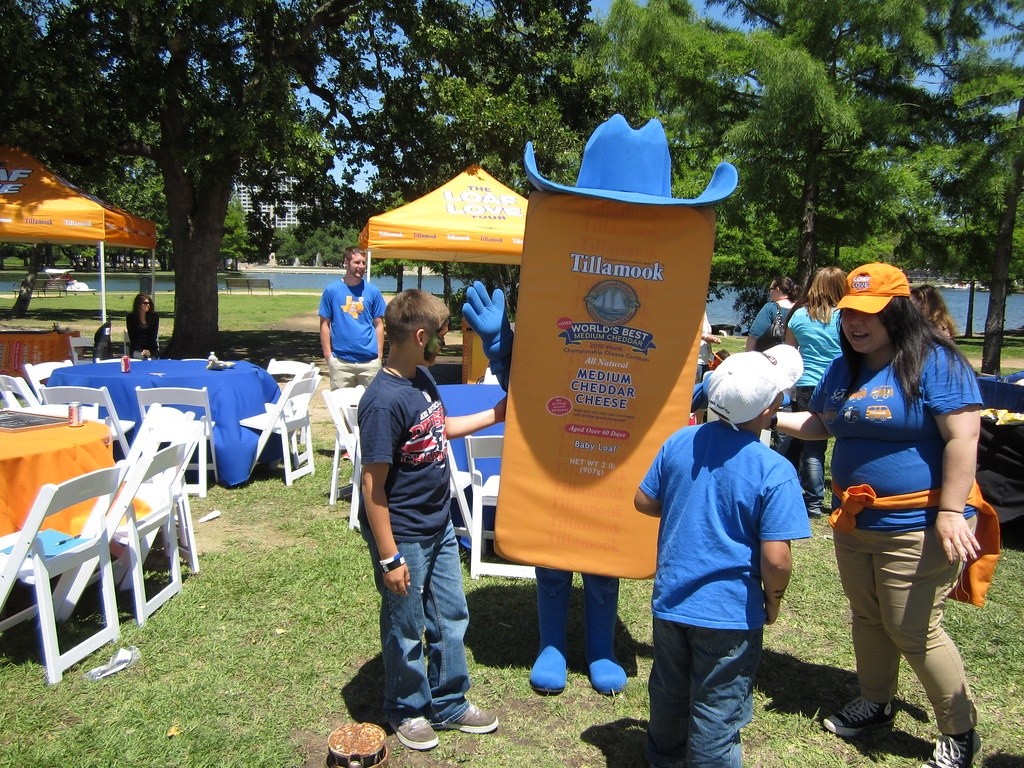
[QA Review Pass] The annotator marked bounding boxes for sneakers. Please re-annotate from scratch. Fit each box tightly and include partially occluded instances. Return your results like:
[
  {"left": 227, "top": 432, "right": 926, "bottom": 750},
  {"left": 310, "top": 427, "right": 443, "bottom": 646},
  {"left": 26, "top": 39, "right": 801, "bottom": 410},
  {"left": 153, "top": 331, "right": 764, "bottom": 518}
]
[
  {"left": 920, "top": 728, "right": 983, "bottom": 768},
  {"left": 387, "top": 713, "right": 439, "bottom": 750},
  {"left": 823, "top": 696, "right": 894, "bottom": 738},
  {"left": 431, "top": 703, "right": 498, "bottom": 734}
]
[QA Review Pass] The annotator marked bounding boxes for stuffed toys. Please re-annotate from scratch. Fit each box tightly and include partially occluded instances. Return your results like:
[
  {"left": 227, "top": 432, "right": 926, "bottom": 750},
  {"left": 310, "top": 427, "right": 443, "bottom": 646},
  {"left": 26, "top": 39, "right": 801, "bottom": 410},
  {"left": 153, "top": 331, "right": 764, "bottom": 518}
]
[{"left": 462, "top": 112, "right": 739, "bottom": 695}]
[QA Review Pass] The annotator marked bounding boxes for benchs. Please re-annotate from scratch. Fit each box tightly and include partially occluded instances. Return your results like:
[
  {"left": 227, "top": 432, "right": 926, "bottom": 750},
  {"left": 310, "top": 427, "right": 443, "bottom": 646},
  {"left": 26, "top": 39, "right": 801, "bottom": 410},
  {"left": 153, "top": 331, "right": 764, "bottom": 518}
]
[
  {"left": 224, "top": 277, "right": 274, "bottom": 296},
  {"left": 13, "top": 279, "right": 69, "bottom": 298}
]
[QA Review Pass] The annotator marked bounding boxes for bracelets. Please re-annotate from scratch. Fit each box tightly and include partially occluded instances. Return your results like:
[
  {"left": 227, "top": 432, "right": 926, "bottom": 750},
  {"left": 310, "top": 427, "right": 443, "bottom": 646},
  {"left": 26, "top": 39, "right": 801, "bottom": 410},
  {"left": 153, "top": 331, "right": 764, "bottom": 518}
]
[
  {"left": 379, "top": 552, "right": 406, "bottom": 572},
  {"left": 938, "top": 509, "right": 964, "bottom": 514},
  {"left": 765, "top": 414, "right": 778, "bottom": 431}
]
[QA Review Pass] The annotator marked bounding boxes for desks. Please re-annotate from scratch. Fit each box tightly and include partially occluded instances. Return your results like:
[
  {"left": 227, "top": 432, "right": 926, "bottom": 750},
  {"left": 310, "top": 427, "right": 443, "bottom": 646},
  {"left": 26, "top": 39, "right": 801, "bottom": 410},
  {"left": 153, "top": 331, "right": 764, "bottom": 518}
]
[
  {"left": 0, "top": 329, "right": 81, "bottom": 377},
  {"left": 425, "top": 382, "right": 516, "bottom": 491},
  {"left": 54, "top": 361, "right": 279, "bottom": 489},
  {"left": 46, "top": 268, "right": 75, "bottom": 293},
  {"left": 1, "top": 415, "right": 114, "bottom": 563}
]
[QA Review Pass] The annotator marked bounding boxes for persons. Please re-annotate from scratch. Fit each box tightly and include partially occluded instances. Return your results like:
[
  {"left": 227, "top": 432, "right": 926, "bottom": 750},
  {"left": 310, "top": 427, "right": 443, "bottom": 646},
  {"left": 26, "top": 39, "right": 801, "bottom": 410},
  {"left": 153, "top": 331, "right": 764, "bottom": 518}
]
[
  {"left": 126, "top": 293, "right": 159, "bottom": 359},
  {"left": 764, "top": 262, "right": 999, "bottom": 768},
  {"left": 634, "top": 345, "right": 811, "bottom": 768},
  {"left": 54, "top": 273, "right": 73, "bottom": 281},
  {"left": 318, "top": 247, "right": 387, "bottom": 461},
  {"left": 692, "top": 267, "right": 851, "bottom": 517},
  {"left": 909, "top": 285, "right": 955, "bottom": 343},
  {"left": 359, "top": 289, "right": 507, "bottom": 752}
]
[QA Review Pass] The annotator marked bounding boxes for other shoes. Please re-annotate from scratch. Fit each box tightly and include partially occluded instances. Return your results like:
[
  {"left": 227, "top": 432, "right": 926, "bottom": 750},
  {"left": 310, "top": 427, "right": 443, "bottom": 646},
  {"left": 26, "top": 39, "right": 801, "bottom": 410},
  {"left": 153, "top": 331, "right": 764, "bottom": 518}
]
[{"left": 341, "top": 450, "right": 350, "bottom": 459}]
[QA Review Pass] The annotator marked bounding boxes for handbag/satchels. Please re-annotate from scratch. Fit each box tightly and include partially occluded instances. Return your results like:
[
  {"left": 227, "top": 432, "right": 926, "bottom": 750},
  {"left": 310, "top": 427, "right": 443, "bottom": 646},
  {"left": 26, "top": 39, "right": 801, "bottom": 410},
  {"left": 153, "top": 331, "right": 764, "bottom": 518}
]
[{"left": 756, "top": 300, "right": 785, "bottom": 351}]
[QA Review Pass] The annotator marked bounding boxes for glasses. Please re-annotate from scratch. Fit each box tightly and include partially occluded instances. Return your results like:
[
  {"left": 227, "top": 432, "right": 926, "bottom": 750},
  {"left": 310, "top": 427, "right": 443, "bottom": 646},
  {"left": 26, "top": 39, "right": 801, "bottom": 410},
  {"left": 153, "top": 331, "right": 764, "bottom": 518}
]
[{"left": 143, "top": 302, "right": 151, "bottom": 305}]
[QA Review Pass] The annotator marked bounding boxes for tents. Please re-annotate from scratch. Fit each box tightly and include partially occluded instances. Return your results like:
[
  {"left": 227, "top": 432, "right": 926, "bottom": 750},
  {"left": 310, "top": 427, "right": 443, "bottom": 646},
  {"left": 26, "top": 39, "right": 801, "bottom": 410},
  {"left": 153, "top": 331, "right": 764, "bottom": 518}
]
[
  {"left": 0, "top": 135, "right": 156, "bottom": 324},
  {"left": 356, "top": 164, "right": 530, "bottom": 283}
]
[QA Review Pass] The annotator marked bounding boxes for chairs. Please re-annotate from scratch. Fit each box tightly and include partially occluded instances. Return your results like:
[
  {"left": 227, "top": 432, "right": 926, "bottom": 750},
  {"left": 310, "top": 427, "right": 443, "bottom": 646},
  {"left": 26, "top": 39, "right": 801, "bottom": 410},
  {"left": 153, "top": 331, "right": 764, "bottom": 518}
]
[{"left": 0, "top": 332, "right": 772, "bottom": 683}]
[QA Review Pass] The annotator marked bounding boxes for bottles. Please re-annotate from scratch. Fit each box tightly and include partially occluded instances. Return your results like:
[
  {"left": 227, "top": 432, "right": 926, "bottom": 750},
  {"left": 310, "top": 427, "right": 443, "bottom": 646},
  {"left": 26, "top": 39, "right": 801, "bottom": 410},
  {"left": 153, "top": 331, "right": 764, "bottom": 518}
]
[{"left": 208, "top": 352, "right": 217, "bottom": 364}]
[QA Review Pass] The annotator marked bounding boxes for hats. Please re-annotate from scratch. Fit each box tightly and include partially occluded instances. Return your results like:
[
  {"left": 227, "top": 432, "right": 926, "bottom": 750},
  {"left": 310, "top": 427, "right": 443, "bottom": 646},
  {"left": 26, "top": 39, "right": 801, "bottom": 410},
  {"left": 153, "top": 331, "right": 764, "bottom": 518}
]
[
  {"left": 836, "top": 262, "right": 911, "bottom": 314},
  {"left": 708, "top": 342, "right": 804, "bottom": 431}
]
